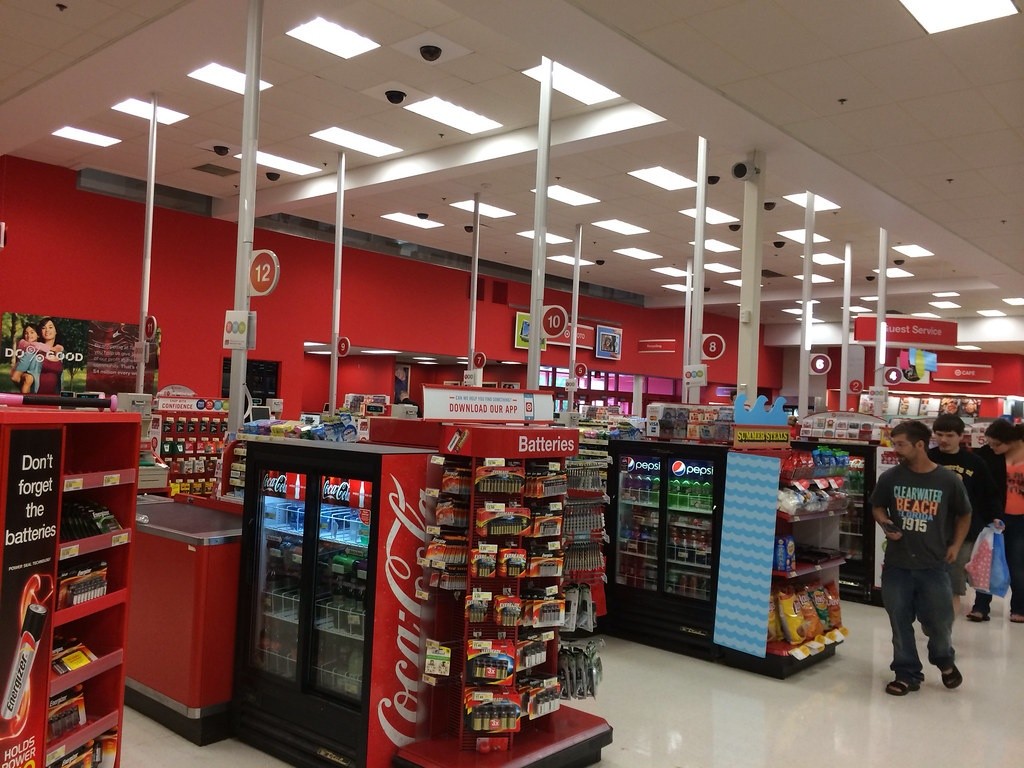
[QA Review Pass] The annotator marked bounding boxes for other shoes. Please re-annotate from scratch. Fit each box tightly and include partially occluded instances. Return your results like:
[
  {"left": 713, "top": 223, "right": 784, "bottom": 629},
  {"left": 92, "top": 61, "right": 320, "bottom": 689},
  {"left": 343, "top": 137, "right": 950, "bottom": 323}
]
[
  {"left": 966, "top": 610, "right": 990, "bottom": 621},
  {"left": 1010, "top": 613, "right": 1024, "bottom": 622}
]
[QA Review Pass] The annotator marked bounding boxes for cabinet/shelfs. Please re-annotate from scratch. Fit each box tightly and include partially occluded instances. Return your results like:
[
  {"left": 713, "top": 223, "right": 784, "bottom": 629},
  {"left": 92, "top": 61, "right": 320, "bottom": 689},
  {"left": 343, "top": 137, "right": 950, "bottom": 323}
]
[
  {"left": 578, "top": 420, "right": 851, "bottom": 680},
  {"left": 0, "top": 388, "right": 581, "bottom": 768},
  {"left": 791, "top": 440, "right": 900, "bottom": 607}
]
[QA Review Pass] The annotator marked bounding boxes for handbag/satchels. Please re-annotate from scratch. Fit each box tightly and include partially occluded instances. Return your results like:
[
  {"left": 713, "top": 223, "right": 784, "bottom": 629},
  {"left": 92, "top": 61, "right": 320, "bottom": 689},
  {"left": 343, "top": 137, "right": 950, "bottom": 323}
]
[{"left": 965, "top": 518, "right": 1011, "bottom": 598}]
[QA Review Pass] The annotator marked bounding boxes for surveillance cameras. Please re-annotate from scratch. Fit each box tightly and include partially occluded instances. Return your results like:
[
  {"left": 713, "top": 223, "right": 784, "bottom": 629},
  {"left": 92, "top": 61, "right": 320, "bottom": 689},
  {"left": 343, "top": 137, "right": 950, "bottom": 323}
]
[
  {"left": 265, "top": 172, "right": 280, "bottom": 181},
  {"left": 419, "top": 45, "right": 442, "bottom": 61},
  {"left": 729, "top": 225, "right": 742, "bottom": 231},
  {"left": 704, "top": 287, "right": 711, "bottom": 292},
  {"left": 708, "top": 176, "right": 720, "bottom": 184},
  {"left": 464, "top": 226, "right": 473, "bottom": 233},
  {"left": 384, "top": 90, "right": 406, "bottom": 104},
  {"left": 866, "top": 276, "right": 875, "bottom": 282},
  {"left": 417, "top": 213, "right": 429, "bottom": 220},
  {"left": 773, "top": 242, "right": 785, "bottom": 248},
  {"left": 595, "top": 260, "right": 604, "bottom": 265},
  {"left": 213, "top": 145, "right": 230, "bottom": 156},
  {"left": 893, "top": 260, "right": 905, "bottom": 265},
  {"left": 764, "top": 202, "right": 776, "bottom": 211}
]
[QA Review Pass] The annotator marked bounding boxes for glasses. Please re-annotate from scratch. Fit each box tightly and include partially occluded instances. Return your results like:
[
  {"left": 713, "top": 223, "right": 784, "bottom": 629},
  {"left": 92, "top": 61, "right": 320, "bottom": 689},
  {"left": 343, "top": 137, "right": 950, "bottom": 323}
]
[{"left": 986, "top": 441, "right": 1003, "bottom": 447}]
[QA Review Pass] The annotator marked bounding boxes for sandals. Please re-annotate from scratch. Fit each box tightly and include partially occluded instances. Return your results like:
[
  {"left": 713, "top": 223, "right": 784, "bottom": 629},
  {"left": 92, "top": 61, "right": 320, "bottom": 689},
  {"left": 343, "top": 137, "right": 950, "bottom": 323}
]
[
  {"left": 940, "top": 663, "right": 962, "bottom": 688},
  {"left": 886, "top": 678, "right": 920, "bottom": 696}
]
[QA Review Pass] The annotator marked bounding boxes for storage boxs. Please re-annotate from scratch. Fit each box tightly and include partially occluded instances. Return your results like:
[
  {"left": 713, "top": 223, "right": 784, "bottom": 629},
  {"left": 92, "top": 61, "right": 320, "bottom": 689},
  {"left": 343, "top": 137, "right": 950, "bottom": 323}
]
[
  {"left": 587, "top": 405, "right": 620, "bottom": 419},
  {"left": 645, "top": 402, "right": 735, "bottom": 441},
  {"left": 800, "top": 417, "right": 887, "bottom": 440}
]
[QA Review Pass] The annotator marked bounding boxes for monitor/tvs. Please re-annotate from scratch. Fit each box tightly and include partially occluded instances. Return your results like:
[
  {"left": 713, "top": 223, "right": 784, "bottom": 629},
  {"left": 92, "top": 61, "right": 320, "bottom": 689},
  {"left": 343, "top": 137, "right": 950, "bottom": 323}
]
[{"left": 250, "top": 406, "right": 271, "bottom": 422}]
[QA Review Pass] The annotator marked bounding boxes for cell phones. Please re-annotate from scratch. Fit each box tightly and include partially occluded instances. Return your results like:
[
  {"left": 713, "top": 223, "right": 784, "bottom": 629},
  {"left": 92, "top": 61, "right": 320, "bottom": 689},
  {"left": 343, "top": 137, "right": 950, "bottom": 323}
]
[{"left": 883, "top": 522, "right": 902, "bottom": 533}]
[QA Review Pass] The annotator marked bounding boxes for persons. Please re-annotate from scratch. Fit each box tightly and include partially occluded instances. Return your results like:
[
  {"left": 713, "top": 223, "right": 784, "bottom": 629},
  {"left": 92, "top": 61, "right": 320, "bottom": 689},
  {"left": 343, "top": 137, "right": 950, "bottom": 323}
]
[
  {"left": 10, "top": 318, "right": 65, "bottom": 394},
  {"left": 967, "top": 418, "right": 1024, "bottom": 622},
  {"left": 927, "top": 413, "right": 993, "bottom": 617},
  {"left": 869, "top": 420, "right": 972, "bottom": 696}
]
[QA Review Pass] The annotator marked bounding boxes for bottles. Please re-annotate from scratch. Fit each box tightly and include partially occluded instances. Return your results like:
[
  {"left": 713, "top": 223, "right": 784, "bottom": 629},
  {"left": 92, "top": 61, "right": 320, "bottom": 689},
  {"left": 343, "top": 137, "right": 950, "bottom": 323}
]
[
  {"left": 259, "top": 499, "right": 370, "bottom": 700},
  {"left": 839, "top": 470, "right": 864, "bottom": 576},
  {"left": 620, "top": 472, "right": 712, "bottom": 600}
]
